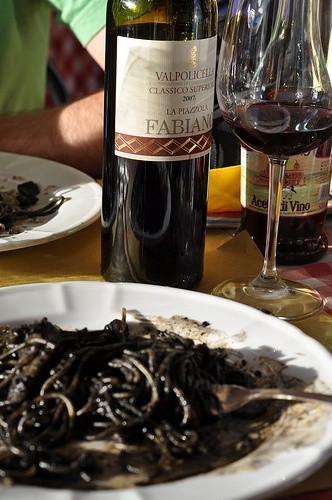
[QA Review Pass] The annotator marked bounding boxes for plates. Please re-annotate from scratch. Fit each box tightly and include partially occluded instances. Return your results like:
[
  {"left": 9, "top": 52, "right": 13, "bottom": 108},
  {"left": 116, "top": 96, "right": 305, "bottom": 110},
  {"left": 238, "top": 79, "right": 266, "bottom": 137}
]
[
  {"left": 0, "top": 282, "right": 332, "bottom": 500},
  {"left": 0, "top": 151, "right": 101, "bottom": 253}
]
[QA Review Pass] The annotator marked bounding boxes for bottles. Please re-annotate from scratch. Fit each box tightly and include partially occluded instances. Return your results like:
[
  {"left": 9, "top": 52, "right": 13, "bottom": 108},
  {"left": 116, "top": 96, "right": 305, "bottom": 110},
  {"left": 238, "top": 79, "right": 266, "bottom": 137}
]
[
  {"left": 240, "top": 0, "right": 332, "bottom": 264},
  {"left": 102, "top": 0, "right": 218, "bottom": 290}
]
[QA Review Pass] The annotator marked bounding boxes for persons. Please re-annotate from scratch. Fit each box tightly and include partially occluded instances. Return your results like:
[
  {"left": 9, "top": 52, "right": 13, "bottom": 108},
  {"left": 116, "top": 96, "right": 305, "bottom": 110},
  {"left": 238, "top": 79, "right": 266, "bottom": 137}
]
[{"left": 0, "top": 0, "right": 108, "bottom": 180}]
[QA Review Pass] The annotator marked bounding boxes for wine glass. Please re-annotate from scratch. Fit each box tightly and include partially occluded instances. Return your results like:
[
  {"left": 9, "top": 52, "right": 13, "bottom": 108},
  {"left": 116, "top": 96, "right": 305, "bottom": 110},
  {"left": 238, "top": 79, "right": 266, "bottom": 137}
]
[{"left": 213, "top": 0, "right": 332, "bottom": 322}]
